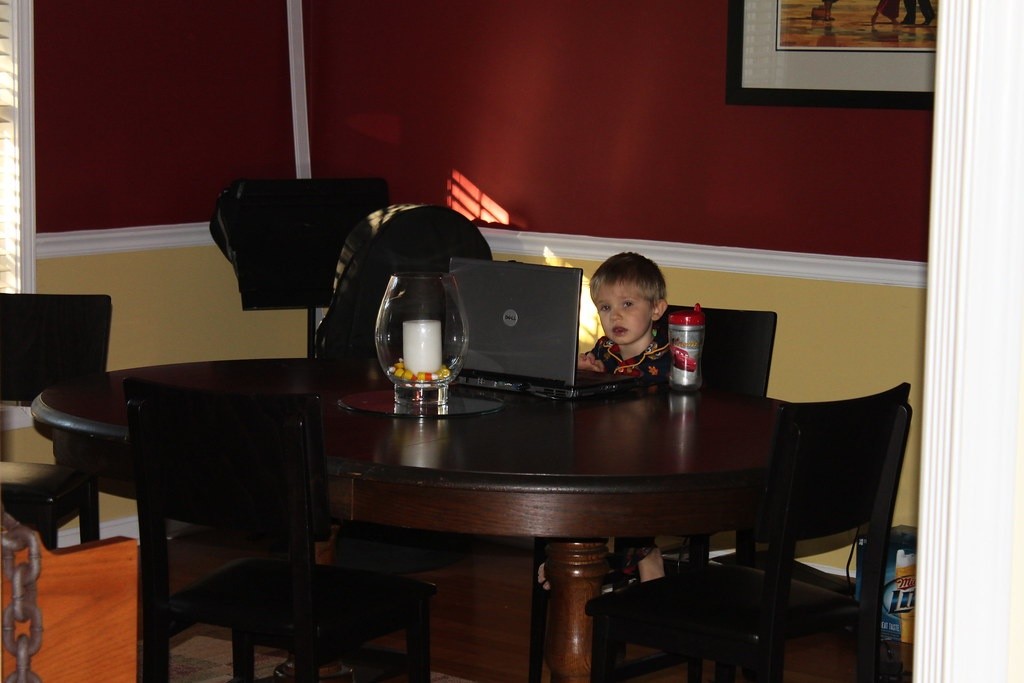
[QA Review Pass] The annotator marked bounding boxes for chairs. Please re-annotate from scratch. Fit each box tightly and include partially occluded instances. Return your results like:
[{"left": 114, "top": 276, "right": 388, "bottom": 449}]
[
  {"left": 651, "top": 302, "right": 779, "bottom": 400},
  {"left": 124, "top": 377, "right": 438, "bottom": 682},
  {"left": 0, "top": 291, "right": 115, "bottom": 551},
  {"left": 583, "top": 382, "right": 912, "bottom": 682}
]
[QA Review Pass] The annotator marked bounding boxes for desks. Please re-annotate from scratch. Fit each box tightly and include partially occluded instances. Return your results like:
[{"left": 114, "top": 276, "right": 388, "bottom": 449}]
[{"left": 33, "top": 357, "right": 794, "bottom": 682}]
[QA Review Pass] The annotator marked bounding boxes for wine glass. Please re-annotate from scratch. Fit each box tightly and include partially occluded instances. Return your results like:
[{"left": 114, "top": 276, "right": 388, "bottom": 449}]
[{"left": 336, "top": 269, "right": 505, "bottom": 418}]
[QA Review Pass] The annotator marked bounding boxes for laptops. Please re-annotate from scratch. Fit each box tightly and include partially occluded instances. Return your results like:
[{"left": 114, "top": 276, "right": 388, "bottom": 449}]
[{"left": 442, "top": 256, "right": 635, "bottom": 399}]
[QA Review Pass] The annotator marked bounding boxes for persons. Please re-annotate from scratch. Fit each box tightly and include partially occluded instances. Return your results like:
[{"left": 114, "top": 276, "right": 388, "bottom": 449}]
[{"left": 575, "top": 252, "right": 670, "bottom": 382}]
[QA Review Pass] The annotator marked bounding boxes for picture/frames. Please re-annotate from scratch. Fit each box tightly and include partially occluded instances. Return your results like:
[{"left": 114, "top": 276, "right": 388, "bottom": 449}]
[{"left": 725, "top": 0, "right": 938, "bottom": 105}]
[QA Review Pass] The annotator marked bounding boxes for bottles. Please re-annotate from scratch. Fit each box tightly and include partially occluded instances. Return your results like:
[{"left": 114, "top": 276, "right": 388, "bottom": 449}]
[{"left": 669, "top": 303, "right": 704, "bottom": 391}]
